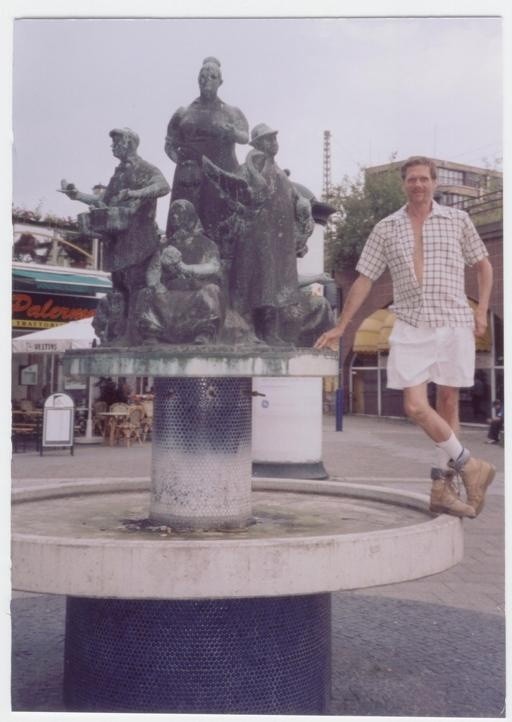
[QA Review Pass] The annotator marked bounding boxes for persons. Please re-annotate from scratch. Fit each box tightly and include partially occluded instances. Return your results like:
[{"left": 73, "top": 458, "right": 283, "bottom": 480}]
[
  {"left": 483, "top": 397, "right": 503, "bottom": 445},
  {"left": 464, "top": 374, "right": 488, "bottom": 418},
  {"left": 95, "top": 377, "right": 134, "bottom": 405},
  {"left": 312, "top": 153, "right": 497, "bottom": 519}
]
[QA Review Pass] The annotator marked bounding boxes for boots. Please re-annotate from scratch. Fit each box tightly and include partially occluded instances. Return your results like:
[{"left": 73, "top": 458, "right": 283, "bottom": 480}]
[{"left": 429, "top": 448, "right": 496, "bottom": 519}]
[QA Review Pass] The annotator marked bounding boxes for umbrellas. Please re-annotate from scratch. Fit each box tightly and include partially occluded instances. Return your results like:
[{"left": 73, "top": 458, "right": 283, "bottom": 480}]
[{"left": 12, "top": 313, "right": 102, "bottom": 439}]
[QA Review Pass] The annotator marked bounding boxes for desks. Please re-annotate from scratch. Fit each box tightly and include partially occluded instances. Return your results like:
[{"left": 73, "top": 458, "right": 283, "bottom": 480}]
[{"left": 14, "top": 411, "right": 42, "bottom": 453}]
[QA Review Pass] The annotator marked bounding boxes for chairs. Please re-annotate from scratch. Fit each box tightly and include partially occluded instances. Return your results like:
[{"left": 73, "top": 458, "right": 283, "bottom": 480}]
[{"left": 21, "top": 399, "right": 146, "bottom": 449}]
